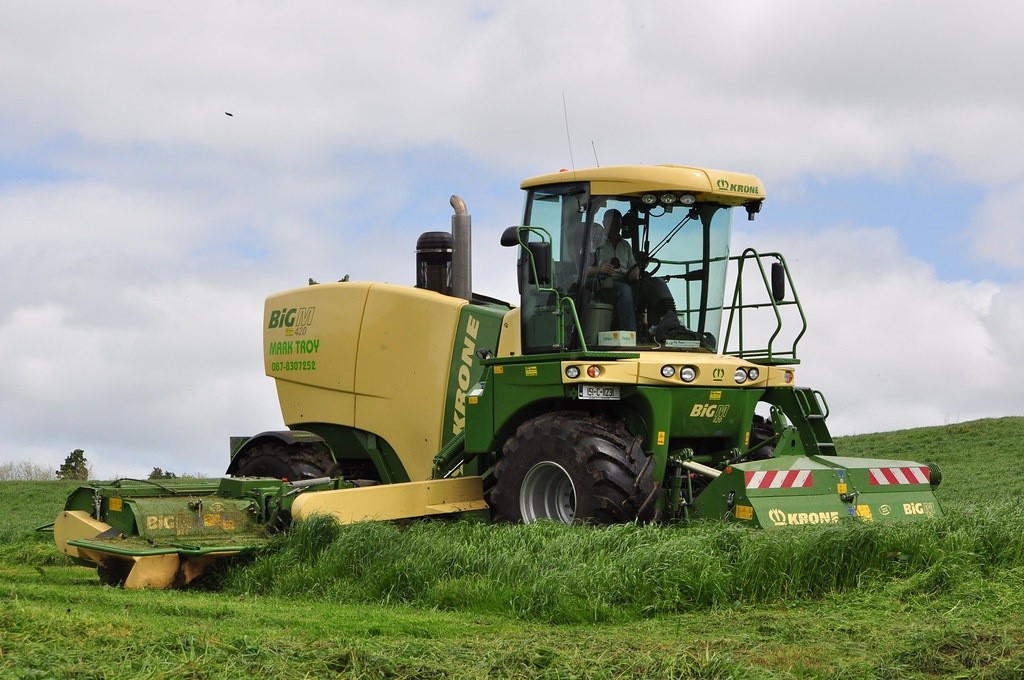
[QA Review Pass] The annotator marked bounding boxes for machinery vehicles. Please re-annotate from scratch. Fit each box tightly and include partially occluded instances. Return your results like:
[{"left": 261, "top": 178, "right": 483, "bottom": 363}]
[{"left": 37, "top": 163, "right": 946, "bottom": 596}]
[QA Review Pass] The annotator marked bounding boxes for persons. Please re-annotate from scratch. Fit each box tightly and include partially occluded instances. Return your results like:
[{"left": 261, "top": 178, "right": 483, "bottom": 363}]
[{"left": 587, "top": 209, "right": 665, "bottom": 342}]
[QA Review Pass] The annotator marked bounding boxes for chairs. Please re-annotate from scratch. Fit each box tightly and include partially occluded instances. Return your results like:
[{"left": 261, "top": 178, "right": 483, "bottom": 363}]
[{"left": 572, "top": 222, "right": 605, "bottom": 298}]
